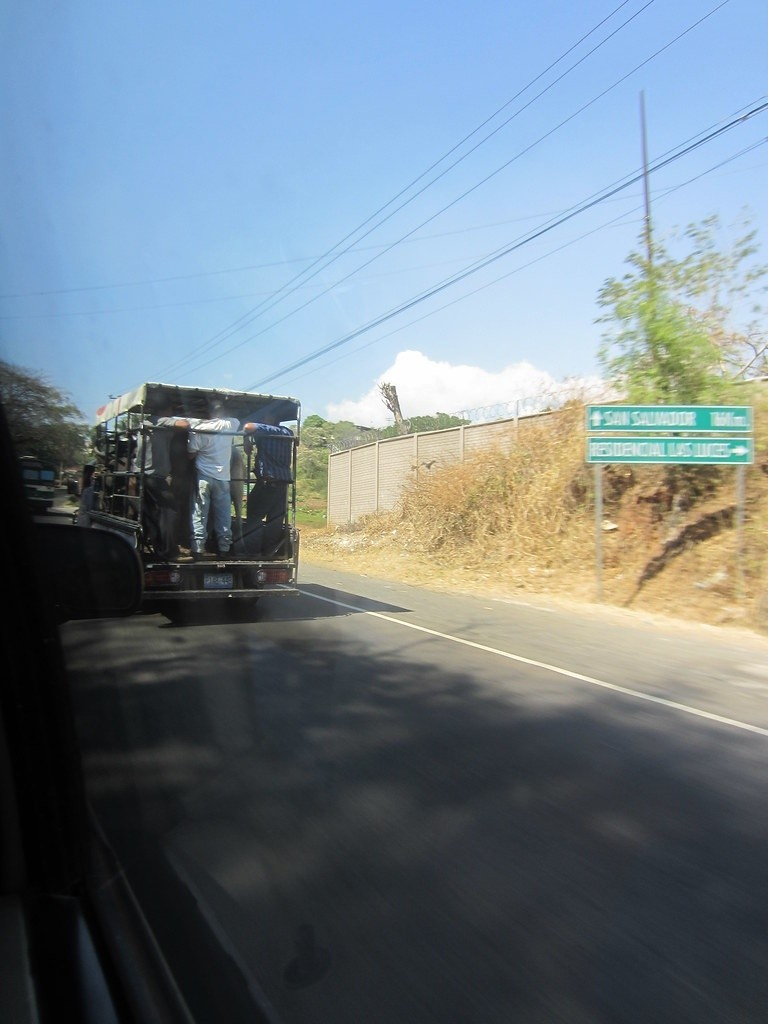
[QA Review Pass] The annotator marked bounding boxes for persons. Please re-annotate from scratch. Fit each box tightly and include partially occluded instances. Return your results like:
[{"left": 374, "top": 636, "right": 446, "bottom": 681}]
[
  {"left": 132, "top": 402, "right": 194, "bottom": 562},
  {"left": 244, "top": 410, "right": 294, "bottom": 559},
  {"left": 187, "top": 399, "right": 240, "bottom": 561}
]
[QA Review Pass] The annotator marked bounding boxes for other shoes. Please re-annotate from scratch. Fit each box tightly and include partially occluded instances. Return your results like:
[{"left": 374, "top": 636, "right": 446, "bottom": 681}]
[
  {"left": 217, "top": 549, "right": 230, "bottom": 559},
  {"left": 190, "top": 551, "right": 202, "bottom": 560}
]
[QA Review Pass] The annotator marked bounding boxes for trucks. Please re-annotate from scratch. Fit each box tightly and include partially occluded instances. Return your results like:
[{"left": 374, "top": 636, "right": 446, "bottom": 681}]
[{"left": 66, "top": 381, "right": 304, "bottom": 611}]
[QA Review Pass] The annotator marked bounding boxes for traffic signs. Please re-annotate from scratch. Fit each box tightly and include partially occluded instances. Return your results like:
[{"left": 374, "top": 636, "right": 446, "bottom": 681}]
[
  {"left": 585, "top": 436, "right": 756, "bottom": 466},
  {"left": 586, "top": 405, "right": 755, "bottom": 432}
]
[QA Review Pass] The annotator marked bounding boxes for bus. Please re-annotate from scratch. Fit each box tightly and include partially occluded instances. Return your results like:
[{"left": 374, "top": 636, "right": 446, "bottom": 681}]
[{"left": 14, "top": 455, "right": 57, "bottom": 514}]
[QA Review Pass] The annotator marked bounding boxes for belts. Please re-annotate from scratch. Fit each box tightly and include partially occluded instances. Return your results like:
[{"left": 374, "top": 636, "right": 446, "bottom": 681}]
[{"left": 264, "top": 481, "right": 288, "bottom": 488}]
